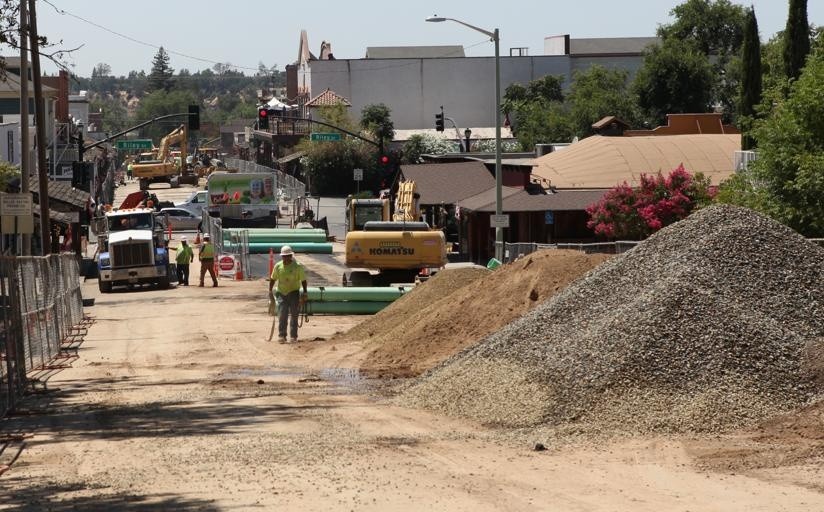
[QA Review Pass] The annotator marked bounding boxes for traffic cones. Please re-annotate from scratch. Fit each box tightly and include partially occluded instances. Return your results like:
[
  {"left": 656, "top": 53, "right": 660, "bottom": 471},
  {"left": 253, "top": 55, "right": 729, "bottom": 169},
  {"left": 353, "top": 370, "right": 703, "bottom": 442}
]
[
  {"left": 234, "top": 262, "right": 244, "bottom": 281},
  {"left": 194, "top": 229, "right": 201, "bottom": 244}
]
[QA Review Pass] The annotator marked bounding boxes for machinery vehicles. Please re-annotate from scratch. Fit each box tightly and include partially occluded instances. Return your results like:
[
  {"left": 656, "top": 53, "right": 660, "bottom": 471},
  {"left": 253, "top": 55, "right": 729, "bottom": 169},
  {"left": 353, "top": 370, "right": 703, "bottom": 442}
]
[
  {"left": 343, "top": 180, "right": 448, "bottom": 286},
  {"left": 131, "top": 124, "right": 239, "bottom": 189},
  {"left": 96, "top": 207, "right": 170, "bottom": 292}
]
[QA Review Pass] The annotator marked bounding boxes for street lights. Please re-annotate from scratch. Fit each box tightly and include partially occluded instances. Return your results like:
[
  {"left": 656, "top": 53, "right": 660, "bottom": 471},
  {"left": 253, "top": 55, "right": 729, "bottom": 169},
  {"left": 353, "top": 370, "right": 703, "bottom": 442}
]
[
  {"left": 76, "top": 123, "right": 85, "bottom": 184},
  {"left": 465, "top": 128, "right": 472, "bottom": 151},
  {"left": 425, "top": 13, "right": 504, "bottom": 261}
]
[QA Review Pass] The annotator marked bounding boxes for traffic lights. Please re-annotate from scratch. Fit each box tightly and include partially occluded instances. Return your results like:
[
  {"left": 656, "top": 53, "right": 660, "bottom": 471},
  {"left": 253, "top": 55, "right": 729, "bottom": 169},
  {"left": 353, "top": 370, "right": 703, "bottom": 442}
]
[
  {"left": 380, "top": 154, "right": 391, "bottom": 176},
  {"left": 259, "top": 108, "right": 282, "bottom": 117},
  {"left": 436, "top": 111, "right": 445, "bottom": 133}
]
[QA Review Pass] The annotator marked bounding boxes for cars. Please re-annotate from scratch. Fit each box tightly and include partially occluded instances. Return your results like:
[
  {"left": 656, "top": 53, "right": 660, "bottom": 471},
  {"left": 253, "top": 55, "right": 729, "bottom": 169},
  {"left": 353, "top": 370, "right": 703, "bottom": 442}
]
[{"left": 156, "top": 207, "right": 203, "bottom": 233}]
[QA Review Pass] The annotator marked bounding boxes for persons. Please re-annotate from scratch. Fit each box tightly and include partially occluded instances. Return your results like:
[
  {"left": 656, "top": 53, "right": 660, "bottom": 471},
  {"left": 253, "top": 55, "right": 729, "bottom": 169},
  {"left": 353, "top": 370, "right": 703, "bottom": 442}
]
[
  {"left": 126, "top": 161, "right": 133, "bottom": 180},
  {"left": 165, "top": 235, "right": 193, "bottom": 285},
  {"left": 269, "top": 245, "right": 307, "bottom": 343},
  {"left": 174, "top": 161, "right": 179, "bottom": 173},
  {"left": 198, "top": 233, "right": 217, "bottom": 287}
]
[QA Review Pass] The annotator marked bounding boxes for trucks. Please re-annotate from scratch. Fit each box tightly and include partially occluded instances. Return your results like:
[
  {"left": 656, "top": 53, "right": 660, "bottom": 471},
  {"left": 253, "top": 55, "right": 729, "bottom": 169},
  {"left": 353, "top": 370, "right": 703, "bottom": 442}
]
[{"left": 174, "top": 171, "right": 280, "bottom": 229}]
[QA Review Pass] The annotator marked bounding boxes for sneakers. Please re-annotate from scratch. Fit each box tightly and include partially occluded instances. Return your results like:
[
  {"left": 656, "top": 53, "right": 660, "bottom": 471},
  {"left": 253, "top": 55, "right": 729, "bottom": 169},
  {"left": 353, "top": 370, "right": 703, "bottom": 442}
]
[
  {"left": 277, "top": 336, "right": 297, "bottom": 343},
  {"left": 176, "top": 280, "right": 218, "bottom": 288}
]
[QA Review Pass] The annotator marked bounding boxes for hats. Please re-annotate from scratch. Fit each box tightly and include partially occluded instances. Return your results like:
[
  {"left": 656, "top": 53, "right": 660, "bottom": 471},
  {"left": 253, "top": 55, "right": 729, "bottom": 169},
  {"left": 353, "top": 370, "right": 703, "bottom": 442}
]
[
  {"left": 180, "top": 236, "right": 187, "bottom": 242},
  {"left": 279, "top": 246, "right": 293, "bottom": 257},
  {"left": 202, "top": 232, "right": 210, "bottom": 239}
]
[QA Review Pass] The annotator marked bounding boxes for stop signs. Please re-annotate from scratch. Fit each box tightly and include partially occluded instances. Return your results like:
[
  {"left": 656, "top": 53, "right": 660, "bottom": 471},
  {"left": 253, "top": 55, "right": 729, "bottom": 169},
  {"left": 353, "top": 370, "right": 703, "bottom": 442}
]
[{"left": 218, "top": 255, "right": 237, "bottom": 275}]
